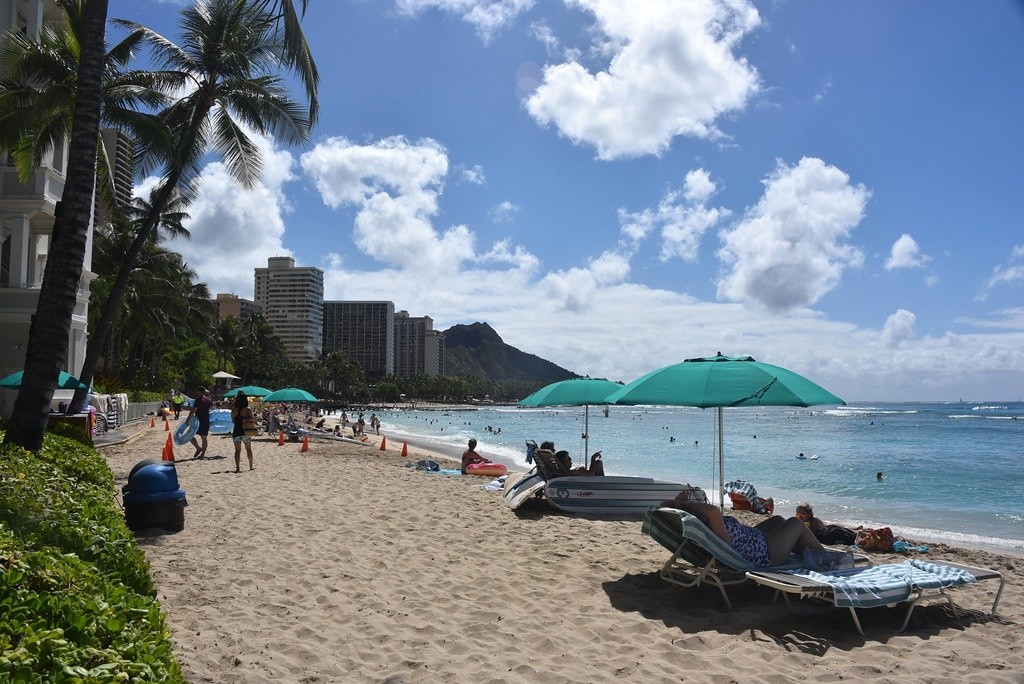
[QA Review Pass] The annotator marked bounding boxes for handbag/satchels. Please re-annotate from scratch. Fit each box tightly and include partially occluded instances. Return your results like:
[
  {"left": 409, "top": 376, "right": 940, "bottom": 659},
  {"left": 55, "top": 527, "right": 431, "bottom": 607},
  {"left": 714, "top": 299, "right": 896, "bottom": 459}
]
[
  {"left": 855, "top": 529, "right": 895, "bottom": 552},
  {"left": 240, "top": 418, "right": 257, "bottom": 436}
]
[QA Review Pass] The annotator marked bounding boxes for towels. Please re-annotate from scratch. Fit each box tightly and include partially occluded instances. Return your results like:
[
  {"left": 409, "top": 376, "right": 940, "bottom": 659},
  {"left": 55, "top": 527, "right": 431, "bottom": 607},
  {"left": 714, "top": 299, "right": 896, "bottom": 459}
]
[
  {"left": 639, "top": 504, "right": 978, "bottom": 608},
  {"left": 268, "top": 414, "right": 296, "bottom": 437},
  {"left": 724, "top": 481, "right": 760, "bottom": 508}
]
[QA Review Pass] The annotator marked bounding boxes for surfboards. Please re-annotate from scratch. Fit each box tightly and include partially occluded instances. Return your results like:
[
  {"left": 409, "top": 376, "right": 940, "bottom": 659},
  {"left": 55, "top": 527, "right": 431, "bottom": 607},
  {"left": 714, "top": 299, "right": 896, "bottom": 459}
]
[
  {"left": 544, "top": 474, "right": 707, "bottom": 514},
  {"left": 503, "top": 473, "right": 545, "bottom": 510}
]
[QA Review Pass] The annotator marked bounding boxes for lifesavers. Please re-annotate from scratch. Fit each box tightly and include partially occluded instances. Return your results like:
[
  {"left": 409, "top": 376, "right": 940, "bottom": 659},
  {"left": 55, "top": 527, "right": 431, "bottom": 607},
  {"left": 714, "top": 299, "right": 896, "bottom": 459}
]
[
  {"left": 466, "top": 463, "right": 507, "bottom": 476},
  {"left": 208, "top": 408, "right": 234, "bottom": 432},
  {"left": 173, "top": 415, "right": 200, "bottom": 444}
]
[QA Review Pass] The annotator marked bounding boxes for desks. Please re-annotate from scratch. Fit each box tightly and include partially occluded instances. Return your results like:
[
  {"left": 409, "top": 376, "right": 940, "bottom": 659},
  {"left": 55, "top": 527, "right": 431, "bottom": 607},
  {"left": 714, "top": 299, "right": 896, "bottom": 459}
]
[{"left": 46, "top": 412, "right": 93, "bottom": 442}]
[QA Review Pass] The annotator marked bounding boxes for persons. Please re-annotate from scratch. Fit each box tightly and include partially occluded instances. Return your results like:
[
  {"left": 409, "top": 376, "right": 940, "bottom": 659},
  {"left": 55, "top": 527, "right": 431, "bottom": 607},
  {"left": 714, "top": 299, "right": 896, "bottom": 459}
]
[
  {"left": 370, "top": 412, "right": 380, "bottom": 436},
  {"left": 661, "top": 491, "right": 841, "bottom": 573},
  {"left": 670, "top": 435, "right": 883, "bottom": 479},
  {"left": 262, "top": 402, "right": 365, "bottom": 436},
  {"left": 186, "top": 386, "right": 215, "bottom": 460},
  {"left": 171, "top": 392, "right": 184, "bottom": 419},
  {"left": 556, "top": 450, "right": 605, "bottom": 476},
  {"left": 461, "top": 438, "right": 492, "bottom": 476},
  {"left": 795, "top": 502, "right": 871, "bottom": 535},
  {"left": 540, "top": 441, "right": 556, "bottom": 454},
  {"left": 231, "top": 394, "right": 255, "bottom": 473},
  {"left": 484, "top": 425, "right": 502, "bottom": 435}
]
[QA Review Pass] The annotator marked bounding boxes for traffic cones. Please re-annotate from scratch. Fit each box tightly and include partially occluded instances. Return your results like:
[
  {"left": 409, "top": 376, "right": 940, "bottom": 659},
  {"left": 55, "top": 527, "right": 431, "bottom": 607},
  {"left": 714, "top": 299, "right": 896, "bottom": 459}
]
[
  {"left": 165, "top": 420, "right": 170, "bottom": 431},
  {"left": 277, "top": 431, "right": 284, "bottom": 446},
  {"left": 378, "top": 437, "right": 386, "bottom": 451},
  {"left": 301, "top": 433, "right": 309, "bottom": 452},
  {"left": 150, "top": 418, "right": 154, "bottom": 428},
  {"left": 162, "top": 431, "right": 176, "bottom": 462},
  {"left": 401, "top": 441, "right": 408, "bottom": 457}
]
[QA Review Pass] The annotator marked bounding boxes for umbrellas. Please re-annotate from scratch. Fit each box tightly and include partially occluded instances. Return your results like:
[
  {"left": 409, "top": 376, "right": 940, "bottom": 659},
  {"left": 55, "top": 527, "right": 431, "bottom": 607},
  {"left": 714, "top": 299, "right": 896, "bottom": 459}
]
[
  {"left": 0, "top": 370, "right": 86, "bottom": 389},
  {"left": 260, "top": 387, "right": 319, "bottom": 411},
  {"left": 222, "top": 385, "right": 272, "bottom": 401},
  {"left": 517, "top": 349, "right": 847, "bottom": 517}
]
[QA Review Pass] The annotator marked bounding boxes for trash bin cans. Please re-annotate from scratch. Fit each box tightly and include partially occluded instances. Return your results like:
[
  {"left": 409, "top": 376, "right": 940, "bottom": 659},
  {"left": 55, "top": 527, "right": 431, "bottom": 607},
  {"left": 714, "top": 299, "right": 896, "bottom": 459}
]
[{"left": 122, "top": 460, "right": 186, "bottom": 533}]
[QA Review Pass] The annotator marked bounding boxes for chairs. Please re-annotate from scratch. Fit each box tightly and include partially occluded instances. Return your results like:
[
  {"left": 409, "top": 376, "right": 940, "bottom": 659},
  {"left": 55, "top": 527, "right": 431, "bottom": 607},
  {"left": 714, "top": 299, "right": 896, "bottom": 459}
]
[
  {"left": 252, "top": 405, "right": 336, "bottom": 443},
  {"left": 535, "top": 448, "right": 570, "bottom": 479},
  {"left": 744, "top": 558, "right": 1005, "bottom": 638},
  {"left": 641, "top": 505, "right": 870, "bottom": 613},
  {"left": 526, "top": 440, "right": 552, "bottom": 482}
]
